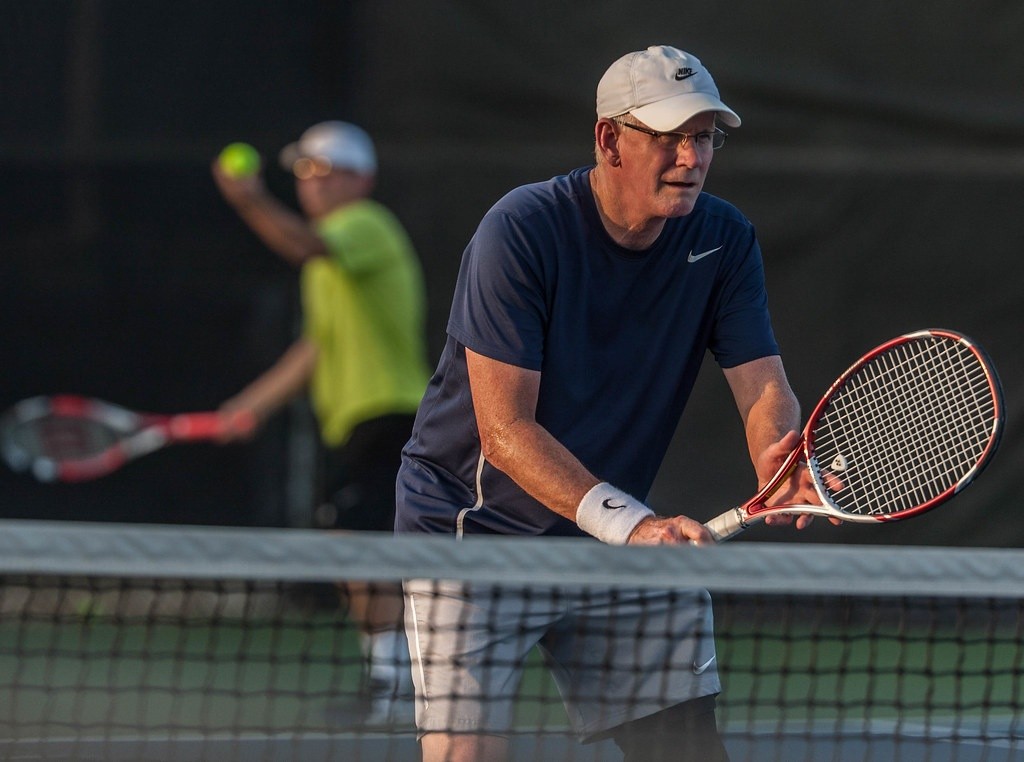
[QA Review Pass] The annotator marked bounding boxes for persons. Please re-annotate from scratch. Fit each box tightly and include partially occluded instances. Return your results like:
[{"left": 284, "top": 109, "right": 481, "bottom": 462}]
[
  {"left": 220, "top": 119, "right": 437, "bottom": 727},
  {"left": 393, "top": 44, "right": 844, "bottom": 761}
]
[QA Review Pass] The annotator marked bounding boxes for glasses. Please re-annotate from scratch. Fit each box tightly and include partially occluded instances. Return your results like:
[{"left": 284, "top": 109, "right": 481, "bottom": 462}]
[{"left": 614, "top": 119, "right": 728, "bottom": 150}]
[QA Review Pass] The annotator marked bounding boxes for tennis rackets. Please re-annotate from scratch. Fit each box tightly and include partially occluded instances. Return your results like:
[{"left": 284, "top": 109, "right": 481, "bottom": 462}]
[
  {"left": 1, "top": 394, "right": 253, "bottom": 482},
  {"left": 685, "top": 327, "right": 1008, "bottom": 548}
]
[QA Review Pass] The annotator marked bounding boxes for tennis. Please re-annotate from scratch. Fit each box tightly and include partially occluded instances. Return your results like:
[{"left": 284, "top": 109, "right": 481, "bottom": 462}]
[{"left": 219, "top": 142, "right": 261, "bottom": 182}]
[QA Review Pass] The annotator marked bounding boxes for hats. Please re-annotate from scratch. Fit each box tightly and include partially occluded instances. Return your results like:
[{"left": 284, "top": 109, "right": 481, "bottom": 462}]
[
  {"left": 597, "top": 45, "right": 741, "bottom": 132},
  {"left": 281, "top": 121, "right": 377, "bottom": 174}
]
